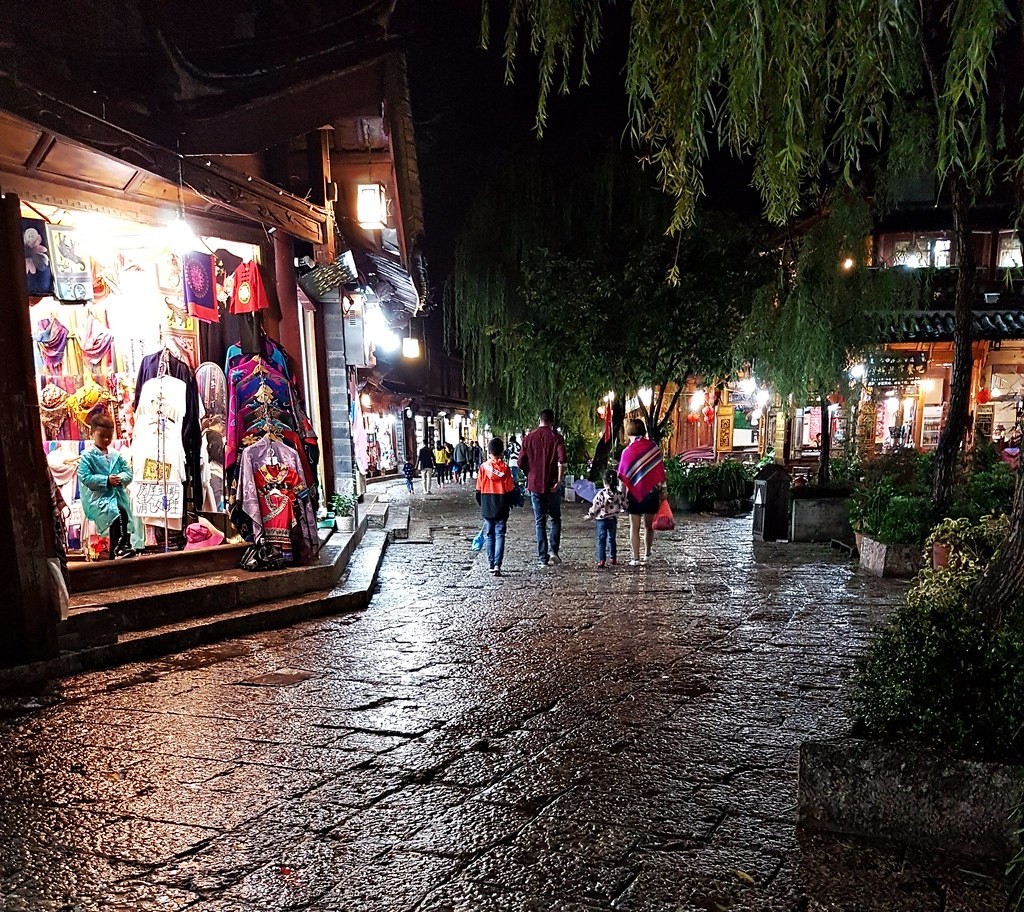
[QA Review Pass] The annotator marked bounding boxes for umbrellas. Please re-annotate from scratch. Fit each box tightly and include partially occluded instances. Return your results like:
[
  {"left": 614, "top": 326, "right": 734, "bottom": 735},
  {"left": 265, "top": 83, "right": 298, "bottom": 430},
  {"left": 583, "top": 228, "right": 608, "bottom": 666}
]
[{"left": 472, "top": 521, "right": 486, "bottom": 551}]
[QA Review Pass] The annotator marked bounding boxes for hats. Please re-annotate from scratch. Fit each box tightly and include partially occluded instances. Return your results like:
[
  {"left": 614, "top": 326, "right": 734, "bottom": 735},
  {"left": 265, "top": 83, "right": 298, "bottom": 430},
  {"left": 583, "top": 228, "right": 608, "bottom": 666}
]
[{"left": 510, "top": 436, "right": 516, "bottom": 442}]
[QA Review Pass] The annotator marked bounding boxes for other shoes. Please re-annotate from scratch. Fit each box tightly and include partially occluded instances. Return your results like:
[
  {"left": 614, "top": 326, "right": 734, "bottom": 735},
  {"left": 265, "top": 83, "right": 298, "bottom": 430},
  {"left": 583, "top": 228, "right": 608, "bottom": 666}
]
[
  {"left": 551, "top": 552, "right": 562, "bottom": 564},
  {"left": 492, "top": 566, "right": 501, "bottom": 575},
  {"left": 629, "top": 560, "right": 639, "bottom": 566},
  {"left": 643, "top": 553, "right": 653, "bottom": 561},
  {"left": 596, "top": 560, "right": 606, "bottom": 566},
  {"left": 608, "top": 558, "right": 616, "bottom": 563}
]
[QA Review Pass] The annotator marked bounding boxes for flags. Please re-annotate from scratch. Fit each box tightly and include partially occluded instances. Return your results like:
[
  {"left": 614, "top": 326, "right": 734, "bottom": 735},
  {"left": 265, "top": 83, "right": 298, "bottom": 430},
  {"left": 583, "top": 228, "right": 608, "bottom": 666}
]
[{"left": 605, "top": 402, "right": 612, "bottom": 442}]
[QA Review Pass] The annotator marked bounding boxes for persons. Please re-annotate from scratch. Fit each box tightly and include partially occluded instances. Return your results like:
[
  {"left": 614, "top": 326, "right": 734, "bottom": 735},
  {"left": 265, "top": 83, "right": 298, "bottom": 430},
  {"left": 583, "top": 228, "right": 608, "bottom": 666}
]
[
  {"left": 517, "top": 409, "right": 566, "bottom": 569},
  {"left": 808, "top": 433, "right": 821, "bottom": 446},
  {"left": 475, "top": 438, "right": 518, "bottom": 575},
  {"left": 432, "top": 437, "right": 483, "bottom": 489},
  {"left": 507, "top": 436, "right": 521, "bottom": 485},
  {"left": 618, "top": 419, "right": 666, "bottom": 566},
  {"left": 583, "top": 469, "right": 629, "bottom": 567},
  {"left": 402, "top": 457, "right": 414, "bottom": 495},
  {"left": 78, "top": 413, "right": 136, "bottom": 559},
  {"left": 415, "top": 439, "right": 436, "bottom": 494}
]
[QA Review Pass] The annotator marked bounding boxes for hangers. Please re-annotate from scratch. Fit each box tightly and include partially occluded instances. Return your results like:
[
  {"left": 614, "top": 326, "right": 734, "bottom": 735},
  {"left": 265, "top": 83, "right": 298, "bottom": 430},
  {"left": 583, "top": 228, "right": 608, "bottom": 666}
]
[
  {"left": 267, "top": 483, "right": 285, "bottom": 501},
  {"left": 255, "top": 394, "right": 272, "bottom": 406},
  {"left": 254, "top": 381, "right": 273, "bottom": 398},
  {"left": 253, "top": 362, "right": 270, "bottom": 377},
  {"left": 250, "top": 352, "right": 263, "bottom": 363},
  {"left": 261, "top": 448, "right": 285, "bottom": 473},
  {"left": 261, "top": 429, "right": 279, "bottom": 445},
  {"left": 258, "top": 402, "right": 275, "bottom": 414},
  {"left": 260, "top": 418, "right": 278, "bottom": 433},
  {"left": 149, "top": 362, "right": 173, "bottom": 432},
  {"left": 240, "top": 252, "right": 252, "bottom": 265},
  {"left": 258, "top": 404, "right": 275, "bottom": 412}
]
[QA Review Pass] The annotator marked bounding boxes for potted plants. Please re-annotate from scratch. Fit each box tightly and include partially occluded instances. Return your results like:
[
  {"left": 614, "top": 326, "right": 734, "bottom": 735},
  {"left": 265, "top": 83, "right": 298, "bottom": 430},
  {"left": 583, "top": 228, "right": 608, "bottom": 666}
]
[
  {"left": 922, "top": 516, "right": 979, "bottom": 571},
  {"left": 706, "top": 458, "right": 752, "bottom": 516},
  {"left": 846, "top": 472, "right": 935, "bottom": 575},
  {"left": 666, "top": 471, "right": 706, "bottom": 511},
  {"left": 327, "top": 492, "right": 361, "bottom": 534}
]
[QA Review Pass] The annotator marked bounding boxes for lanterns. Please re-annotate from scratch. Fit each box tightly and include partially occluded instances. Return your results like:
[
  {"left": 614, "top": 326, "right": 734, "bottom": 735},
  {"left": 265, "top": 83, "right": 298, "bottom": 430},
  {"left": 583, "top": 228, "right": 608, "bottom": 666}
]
[
  {"left": 688, "top": 393, "right": 717, "bottom": 426},
  {"left": 827, "top": 390, "right": 845, "bottom": 404},
  {"left": 976, "top": 388, "right": 991, "bottom": 405}
]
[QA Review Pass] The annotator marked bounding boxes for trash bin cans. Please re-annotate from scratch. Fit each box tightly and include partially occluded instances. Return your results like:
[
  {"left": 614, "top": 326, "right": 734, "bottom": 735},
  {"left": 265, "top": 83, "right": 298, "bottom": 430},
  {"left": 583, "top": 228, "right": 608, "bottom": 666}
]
[{"left": 752, "top": 463, "right": 794, "bottom": 542}]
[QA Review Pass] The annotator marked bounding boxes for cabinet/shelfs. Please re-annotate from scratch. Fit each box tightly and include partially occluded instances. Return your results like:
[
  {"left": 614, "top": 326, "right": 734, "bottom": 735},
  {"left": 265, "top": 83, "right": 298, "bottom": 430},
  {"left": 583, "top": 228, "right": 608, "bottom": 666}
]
[{"left": 0, "top": 70, "right": 254, "bottom": 223}]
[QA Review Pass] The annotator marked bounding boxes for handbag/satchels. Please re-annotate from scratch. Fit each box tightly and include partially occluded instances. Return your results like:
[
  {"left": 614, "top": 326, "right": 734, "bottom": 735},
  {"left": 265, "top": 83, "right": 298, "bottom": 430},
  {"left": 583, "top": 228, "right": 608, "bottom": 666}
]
[
  {"left": 652, "top": 498, "right": 676, "bottom": 531},
  {"left": 472, "top": 524, "right": 489, "bottom": 550}
]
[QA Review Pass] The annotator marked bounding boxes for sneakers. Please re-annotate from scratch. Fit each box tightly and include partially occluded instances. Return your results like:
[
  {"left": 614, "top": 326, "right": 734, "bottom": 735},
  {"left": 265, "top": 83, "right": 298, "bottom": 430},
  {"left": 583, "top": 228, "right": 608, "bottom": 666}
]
[{"left": 114, "top": 549, "right": 134, "bottom": 560}]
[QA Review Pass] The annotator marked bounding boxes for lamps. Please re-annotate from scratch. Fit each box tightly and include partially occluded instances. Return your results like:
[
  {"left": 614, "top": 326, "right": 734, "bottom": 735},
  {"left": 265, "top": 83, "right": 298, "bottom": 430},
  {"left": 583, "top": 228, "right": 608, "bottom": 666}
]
[{"left": 356, "top": 179, "right": 388, "bottom": 232}]
